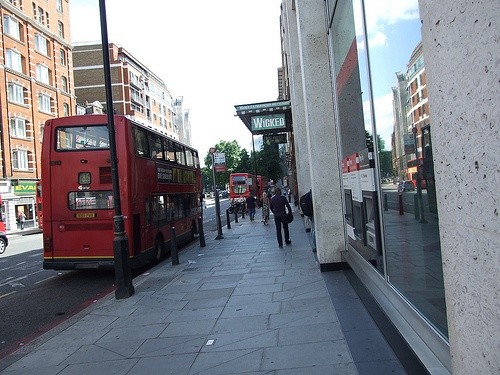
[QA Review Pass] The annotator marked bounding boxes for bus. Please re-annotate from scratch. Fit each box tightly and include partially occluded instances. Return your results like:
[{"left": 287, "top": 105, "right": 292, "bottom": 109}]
[
  {"left": 229, "top": 173, "right": 263, "bottom": 204},
  {"left": 40, "top": 114, "right": 205, "bottom": 272},
  {"left": 420, "top": 123, "right": 438, "bottom": 213},
  {"left": 35, "top": 179, "right": 42, "bottom": 230},
  {"left": 406, "top": 157, "right": 426, "bottom": 189}
]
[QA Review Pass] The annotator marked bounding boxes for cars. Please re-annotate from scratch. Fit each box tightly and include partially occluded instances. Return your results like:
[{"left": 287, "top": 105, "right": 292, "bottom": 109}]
[
  {"left": 217, "top": 191, "right": 228, "bottom": 199},
  {"left": 397, "top": 181, "right": 415, "bottom": 192}
]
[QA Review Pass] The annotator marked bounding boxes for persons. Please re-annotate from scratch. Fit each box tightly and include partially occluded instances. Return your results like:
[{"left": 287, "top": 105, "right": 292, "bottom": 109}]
[
  {"left": 269, "top": 188, "right": 292, "bottom": 248},
  {"left": 19, "top": 212, "right": 26, "bottom": 230},
  {"left": 310, "top": 189, "right": 317, "bottom": 252},
  {"left": 245, "top": 191, "right": 256, "bottom": 222},
  {"left": 262, "top": 193, "right": 269, "bottom": 226}
]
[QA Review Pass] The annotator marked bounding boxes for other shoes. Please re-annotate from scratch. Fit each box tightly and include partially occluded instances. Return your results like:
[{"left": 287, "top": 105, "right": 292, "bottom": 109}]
[
  {"left": 285, "top": 239, "right": 292, "bottom": 245},
  {"left": 278, "top": 242, "right": 283, "bottom": 247}
]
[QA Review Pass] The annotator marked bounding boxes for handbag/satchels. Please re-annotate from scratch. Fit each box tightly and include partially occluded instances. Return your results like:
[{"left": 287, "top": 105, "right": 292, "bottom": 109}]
[{"left": 285, "top": 213, "right": 294, "bottom": 223}]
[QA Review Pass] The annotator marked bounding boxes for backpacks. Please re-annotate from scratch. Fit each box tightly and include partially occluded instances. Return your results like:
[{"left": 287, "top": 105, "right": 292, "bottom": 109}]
[{"left": 300, "top": 192, "right": 313, "bottom": 217}]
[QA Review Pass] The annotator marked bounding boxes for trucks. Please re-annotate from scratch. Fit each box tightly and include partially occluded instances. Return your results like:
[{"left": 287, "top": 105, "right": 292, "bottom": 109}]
[{"left": 0, "top": 194, "right": 8, "bottom": 254}]
